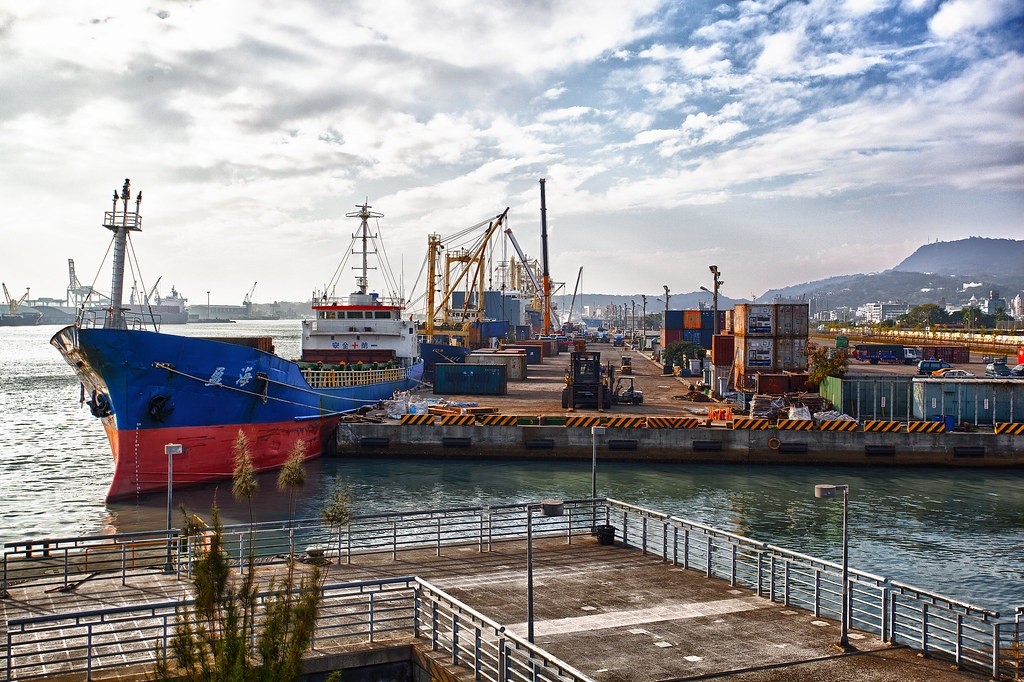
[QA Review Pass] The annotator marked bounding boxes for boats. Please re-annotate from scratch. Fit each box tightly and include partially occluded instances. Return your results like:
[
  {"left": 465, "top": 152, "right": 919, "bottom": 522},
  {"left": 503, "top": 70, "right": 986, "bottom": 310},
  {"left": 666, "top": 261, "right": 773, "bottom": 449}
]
[{"left": 50, "top": 177, "right": 425, "bottom": 503}]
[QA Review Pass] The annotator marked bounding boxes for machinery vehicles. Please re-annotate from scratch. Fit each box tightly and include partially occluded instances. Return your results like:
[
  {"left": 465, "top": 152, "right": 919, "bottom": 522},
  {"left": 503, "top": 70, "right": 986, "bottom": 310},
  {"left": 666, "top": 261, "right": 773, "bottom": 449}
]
[
  {"left": 615, "top": 356, "right": 633, "bottom": 375},
  {"left": 559, "top": 347, "right": 615, "bottom": 412}
]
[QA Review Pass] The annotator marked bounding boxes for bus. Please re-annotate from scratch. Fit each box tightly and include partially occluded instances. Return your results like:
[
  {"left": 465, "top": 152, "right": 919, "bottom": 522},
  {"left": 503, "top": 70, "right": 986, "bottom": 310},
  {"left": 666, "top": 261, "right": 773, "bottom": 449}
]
[{"left": 835, "top": 336, "right": 849, "bottom": 349}]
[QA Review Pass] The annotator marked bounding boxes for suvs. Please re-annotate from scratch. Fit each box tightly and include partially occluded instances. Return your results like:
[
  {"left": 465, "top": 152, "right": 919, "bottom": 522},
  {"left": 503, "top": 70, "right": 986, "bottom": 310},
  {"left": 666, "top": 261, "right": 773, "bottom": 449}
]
[
  {"left": 985, "top": 363, "right": 1011, "bottom": 376},
  {"left": 1009, "top": 363, "right": 1024, "bottom": 376}
]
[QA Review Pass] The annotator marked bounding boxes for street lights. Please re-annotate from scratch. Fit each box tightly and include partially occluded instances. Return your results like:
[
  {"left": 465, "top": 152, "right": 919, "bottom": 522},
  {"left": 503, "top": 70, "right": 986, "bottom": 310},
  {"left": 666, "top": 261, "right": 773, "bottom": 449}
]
[
  {"left": 527, "top": 500, "right": 565, "bottom": 681},
  {"left": 657, "top": 285, "right": 671, "bottom": 310},
  {"left": 607, "top": 302, "right": 631, "bottom": 338},
  {"left": 814, "top": 485, "right": 857, "bottom": 652},
  {"left": 630, "top": 299, "right": 636, "bottom": 332},
  {"left": 637, "top": 295, "right": 647, "bottom": 335},
  {"left": 592, "top": 425, "right": 606, "bottom": 533},
  {"left": 164, "top": 442, "right": 182, "bottom": 576},
  {"left": 700, "top": 264, "right": 725, "bottom": 334}
]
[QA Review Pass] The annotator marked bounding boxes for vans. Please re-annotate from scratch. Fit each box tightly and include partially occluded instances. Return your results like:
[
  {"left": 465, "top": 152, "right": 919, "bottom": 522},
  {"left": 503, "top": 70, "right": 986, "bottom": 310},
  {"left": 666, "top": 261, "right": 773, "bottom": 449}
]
[{"left": 916, "top": 360, "right": 953, "bottom": 374}]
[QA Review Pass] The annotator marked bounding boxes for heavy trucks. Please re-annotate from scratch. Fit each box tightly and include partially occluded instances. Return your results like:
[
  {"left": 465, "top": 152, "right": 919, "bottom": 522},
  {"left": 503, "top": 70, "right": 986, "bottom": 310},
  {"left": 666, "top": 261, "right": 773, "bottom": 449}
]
[
  {"left": 855, "top": 346, "right": 917, "bottom": 366},
  {"left": 912, "top": 347, "right": 970, "bottom": 365}
]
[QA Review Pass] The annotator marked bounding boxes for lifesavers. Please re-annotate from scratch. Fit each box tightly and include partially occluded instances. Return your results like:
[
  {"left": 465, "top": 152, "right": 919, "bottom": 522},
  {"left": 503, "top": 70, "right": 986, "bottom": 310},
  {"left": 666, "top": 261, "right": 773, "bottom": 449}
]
[{"left": 767, "top": 437, "right": 782, "bottom": 451}]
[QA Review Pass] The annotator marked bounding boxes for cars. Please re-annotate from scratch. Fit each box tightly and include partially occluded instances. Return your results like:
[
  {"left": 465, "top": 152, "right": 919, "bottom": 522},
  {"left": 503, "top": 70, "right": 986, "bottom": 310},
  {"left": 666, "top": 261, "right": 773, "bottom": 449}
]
[
  {"left": 950, "top": 369, "right": 976, "bottom": 378},
  {"left": 931, "top": 368, "right": 955, "bottom": 376},
  {"left": 937, "top": 371, "right": 967, "bottom": 379}
]
